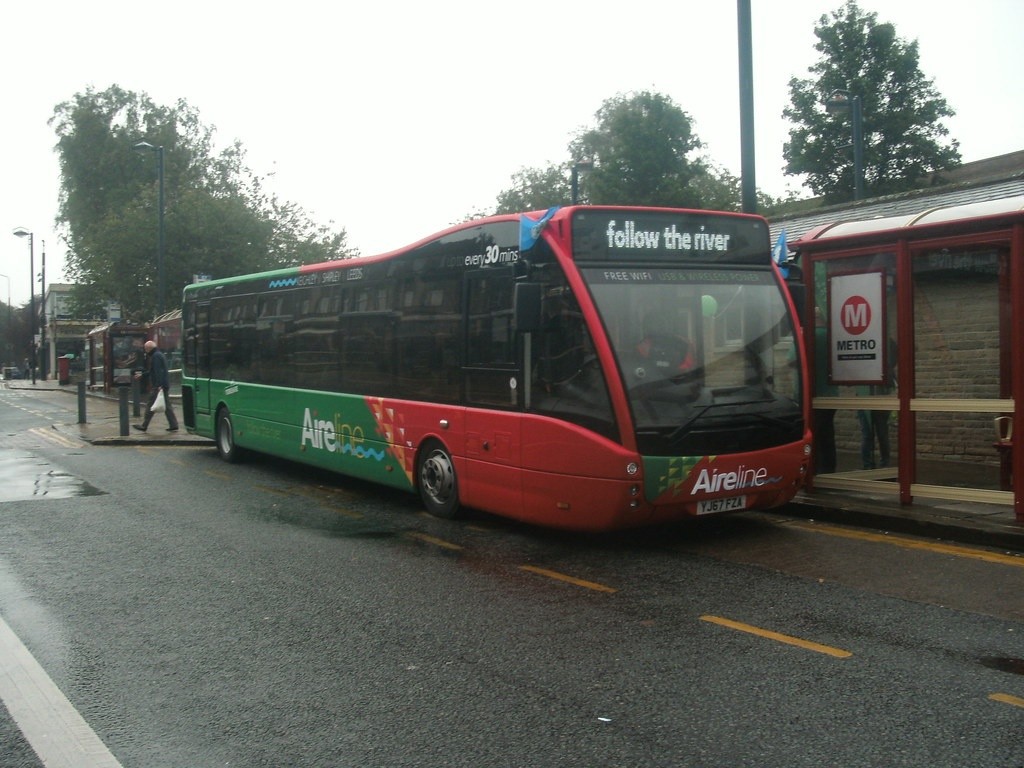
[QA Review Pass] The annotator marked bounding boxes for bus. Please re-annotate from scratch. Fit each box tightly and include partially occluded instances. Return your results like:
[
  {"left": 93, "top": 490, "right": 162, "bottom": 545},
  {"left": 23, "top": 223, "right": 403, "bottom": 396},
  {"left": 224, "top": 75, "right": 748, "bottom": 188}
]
[{"left": 179, "top": 205, "right": 814, "bottom": 536}]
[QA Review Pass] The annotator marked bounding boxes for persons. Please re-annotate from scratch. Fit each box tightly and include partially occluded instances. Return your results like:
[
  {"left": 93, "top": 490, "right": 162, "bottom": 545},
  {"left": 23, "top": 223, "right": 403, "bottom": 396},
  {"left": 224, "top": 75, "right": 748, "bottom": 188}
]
[
  {"left": 132, "top": 341, "right": 179, "bottom": 433},
  {"left": 23, "top": 358, "right": 31, "bottom": 381},
  {"left": 785, "top": 306, "right": 840, "bottom": 476},
  {"left": 854, "top": 335, "right": 899, "bottom": 470}
]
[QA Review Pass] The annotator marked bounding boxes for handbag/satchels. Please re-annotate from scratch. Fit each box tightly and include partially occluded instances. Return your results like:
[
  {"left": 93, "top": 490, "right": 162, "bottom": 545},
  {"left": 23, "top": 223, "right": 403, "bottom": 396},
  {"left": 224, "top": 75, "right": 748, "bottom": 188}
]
[{"left": 151, "top": 389, "right": 166, "bottom": 414}]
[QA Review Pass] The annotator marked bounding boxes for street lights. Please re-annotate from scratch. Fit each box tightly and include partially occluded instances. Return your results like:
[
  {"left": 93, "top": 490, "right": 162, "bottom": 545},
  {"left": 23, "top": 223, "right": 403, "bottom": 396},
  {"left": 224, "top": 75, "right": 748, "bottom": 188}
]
[
  {"left": 14, "top": 227, "right": 36, "bottom": 385},
  {"left": 570, "top": 155, "right": 593, "bottom": 205},
  {"left": 826, "top": 89, "right": 863, "bottom": 201},
  {"left": 129, "top": 137, "right": 164, "bottom": 315}
]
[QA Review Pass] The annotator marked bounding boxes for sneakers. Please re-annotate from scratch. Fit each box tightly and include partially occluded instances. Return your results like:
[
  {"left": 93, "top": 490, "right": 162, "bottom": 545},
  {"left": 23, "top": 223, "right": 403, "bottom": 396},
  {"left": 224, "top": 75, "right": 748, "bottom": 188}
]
[
  {"left": 132, "top": 424, "right": 147, "bottom": 432},
  {"left": 166, "top": 426, "right": 179, "bottom": 432}
]
